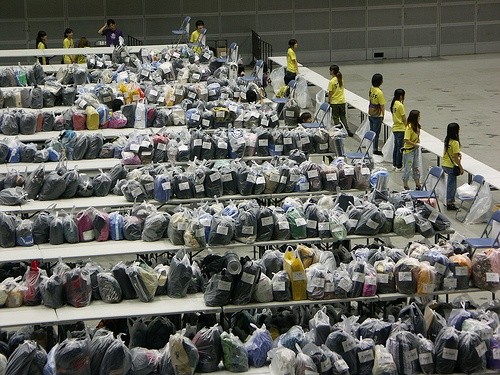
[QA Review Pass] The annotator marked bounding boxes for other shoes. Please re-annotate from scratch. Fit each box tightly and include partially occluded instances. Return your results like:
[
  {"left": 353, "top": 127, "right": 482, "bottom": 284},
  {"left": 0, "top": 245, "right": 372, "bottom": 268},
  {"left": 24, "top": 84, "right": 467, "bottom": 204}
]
[
  {"left": 373, "top": 151, "right": 383, "bottom": 155},
  {"left": 392, "top": 166, "right": 397, "bottom": 171},
  {"left": 416, "top": 185, "right": 421, "bottom": 190},
  {"left": 348, "top": 131, "right": 353, "bottom": 137},
  {"left": 395, "top": 168, "right": 404, "bottom": 173},
  {"left": 404, "top": 186, "right": 410, "bottom": 190},
  {"left": 447, "top": 204, "right": 459, "bottom": 211}
]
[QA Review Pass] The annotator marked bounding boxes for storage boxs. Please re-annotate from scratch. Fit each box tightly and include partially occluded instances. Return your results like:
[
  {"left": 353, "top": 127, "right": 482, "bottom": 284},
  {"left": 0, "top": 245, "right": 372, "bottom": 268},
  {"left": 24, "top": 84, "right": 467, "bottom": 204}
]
[{"left": 217, "top": 47, "right": 229, "bottom": 58}]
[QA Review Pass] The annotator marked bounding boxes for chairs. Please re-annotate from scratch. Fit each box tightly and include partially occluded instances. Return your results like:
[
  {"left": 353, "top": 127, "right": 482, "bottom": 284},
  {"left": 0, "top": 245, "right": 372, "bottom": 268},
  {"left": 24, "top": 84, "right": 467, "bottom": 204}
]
[
  {"left": 215, "top": 43, "right": 240, "bottom": 65},
  {"left": 239, "top": 60, "right": 265, "bottom": 87},
  {"left": 301, "top": 102, "right": 333, "bottom": 129},
  {"left": 188, "top": 28, "right": 208, "bottom": 53},
  {"left": 410, "top": 166, "right": 444, "bottom": 213},
  {"left": 271, "top": 80, "right": 297, "bottom": 119},
  {"left": 455, "top": 175, "right": 485, "bottom": 223},
  {"left": 173, "top": 16, "right": 192, "bottom": 48},
  {"left": 344, "top": 131, "right": 377, "bottom": 168},
  {"left": 464, "top": 210, "right": 500, "bottom": 258}
]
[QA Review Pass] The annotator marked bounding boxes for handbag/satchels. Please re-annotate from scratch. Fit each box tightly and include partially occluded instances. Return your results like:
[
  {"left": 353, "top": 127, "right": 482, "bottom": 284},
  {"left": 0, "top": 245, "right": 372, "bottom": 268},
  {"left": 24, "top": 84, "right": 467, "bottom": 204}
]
[{"left": 0, "top": 48, "right": 500, "bottom": 375}]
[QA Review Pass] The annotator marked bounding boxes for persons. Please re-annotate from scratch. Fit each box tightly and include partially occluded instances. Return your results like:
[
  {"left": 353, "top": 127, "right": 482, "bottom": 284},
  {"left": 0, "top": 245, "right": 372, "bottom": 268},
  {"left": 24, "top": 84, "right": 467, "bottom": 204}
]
[
  {"left": 190, "top": 20, "right": 207, "bottom": 54},
  {"left": 98, "top": 20, "right": 124, "bottom": 47},
  {"left": 286, "top": 39, "right": 298, "bottom": 76},
  {"left": 368, "top": 73, "right": 385, "bottom": 156},
  {"left": 442, "top": 122, "right": 464, "bottom": 211},
  {"left": 246, "top": 90, "right": 259, "bottom": 104},
  {"left": 73, "top": 37, "right": 89, "bottom": 64},
  {"left": 301, "top": 112, "right": 312, "bottom": 124},
  {"left": 276, "top": 75, "right": 294, "bottom": 99},
  {"left": 402, "top": 110, "right": 421, "bottom": 191},
  {"left": 391, "top": 89, "right": 407, "bottom": 172},
  {"left": 35, "top": 31, "right": 51, "bottom": 65},
  {"left": 328, "top": 65, "right": 352, "bottom": 136},
  {"left": 237, "top": 64, "right": 246, "bottom": 77},
  {"left": 62, "top": 29, "right": 75, "bottom": 64}
]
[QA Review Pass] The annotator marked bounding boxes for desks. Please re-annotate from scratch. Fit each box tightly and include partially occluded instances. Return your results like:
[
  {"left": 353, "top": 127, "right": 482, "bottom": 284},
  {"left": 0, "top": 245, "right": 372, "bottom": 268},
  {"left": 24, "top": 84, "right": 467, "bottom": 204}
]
[{"left": 0, "top": 44, "right": 500, "bottom": 375}]
